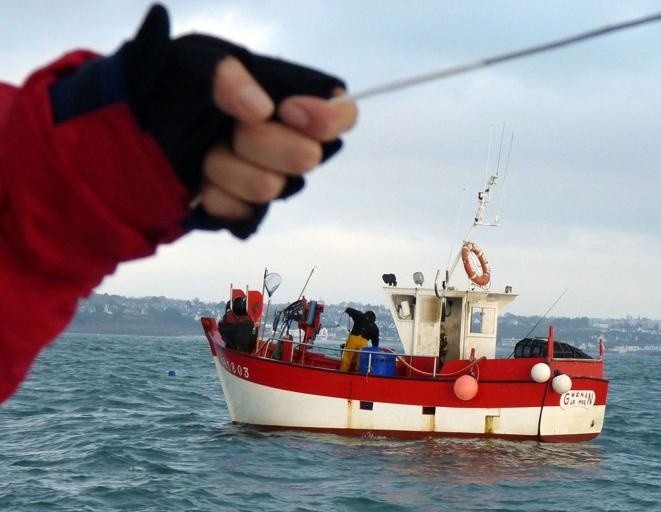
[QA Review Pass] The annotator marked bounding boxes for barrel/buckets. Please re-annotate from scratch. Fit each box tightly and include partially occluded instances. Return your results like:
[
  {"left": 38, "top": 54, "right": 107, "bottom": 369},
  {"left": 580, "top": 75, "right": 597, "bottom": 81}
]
[{"left": 361, "top": 347, "right": 397, "bottom": 376}]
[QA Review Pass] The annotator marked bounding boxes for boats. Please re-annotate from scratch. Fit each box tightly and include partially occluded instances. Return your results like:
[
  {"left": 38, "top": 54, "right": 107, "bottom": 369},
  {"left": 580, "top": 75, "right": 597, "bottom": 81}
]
[{"left": 201, "top": 120, "right": 608, "bottom": 439}]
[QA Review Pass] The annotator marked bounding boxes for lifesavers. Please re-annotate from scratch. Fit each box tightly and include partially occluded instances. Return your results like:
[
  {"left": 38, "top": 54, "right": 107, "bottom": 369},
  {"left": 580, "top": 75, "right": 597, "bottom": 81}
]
[{"left": 462, "top": 242, "right": 490, "bottom": 285}]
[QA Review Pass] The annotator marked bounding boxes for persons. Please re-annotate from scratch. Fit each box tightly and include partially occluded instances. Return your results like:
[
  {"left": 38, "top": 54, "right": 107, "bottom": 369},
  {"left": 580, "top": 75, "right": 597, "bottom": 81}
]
[
  {"left": 338, "top": 306, "right": 380, "bottom": 371},
  {"left": 1, "top": 0, "right": 358, "bottom": 405}
]
